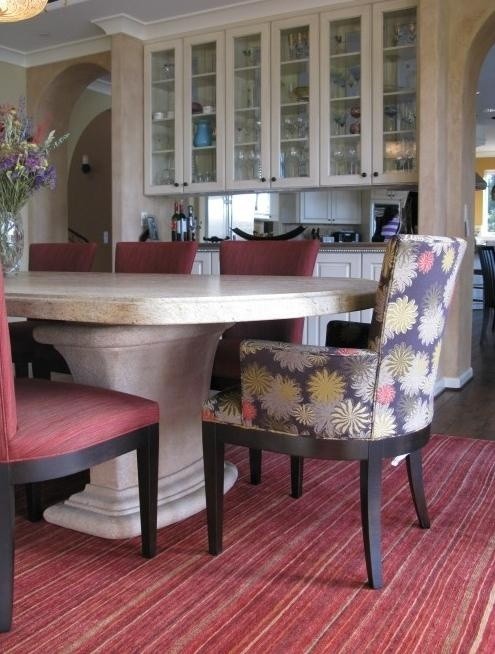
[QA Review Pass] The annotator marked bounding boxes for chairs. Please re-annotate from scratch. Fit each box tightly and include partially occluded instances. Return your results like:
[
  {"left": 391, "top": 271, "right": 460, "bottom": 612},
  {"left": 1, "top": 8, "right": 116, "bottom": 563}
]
[
  {"left": 200, "top": 233, "right": 468, "bottom": 590},
  {"left": 115, "top": 240, "right": 199, "bottom": 275},
  {"left": 210, "top": 237, "right": 324, "bottom": 485},
  {"left": 7, "top": 241, "right": 98, "bottom": 380},
  {"left": 477, "top": 245, "right": 495, "bottom": 348},
  {"left": 0, "top": 256, "right": 160, "bottom": 634}
]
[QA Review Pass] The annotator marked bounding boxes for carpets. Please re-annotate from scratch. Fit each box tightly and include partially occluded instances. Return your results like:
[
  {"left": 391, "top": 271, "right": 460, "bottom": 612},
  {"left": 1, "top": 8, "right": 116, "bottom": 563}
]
[{"left": 0, "top": 433, "right": 494, "bottom": 653}]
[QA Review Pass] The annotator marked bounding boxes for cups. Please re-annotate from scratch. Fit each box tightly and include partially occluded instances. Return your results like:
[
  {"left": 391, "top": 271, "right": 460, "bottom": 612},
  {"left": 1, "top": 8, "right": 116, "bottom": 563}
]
[
  {"left": 203, "top": 106, "right": 212, "bottom": 113},
  {"left": 167, "top": 111, "right": 174, "bottom": 118},
  {"left": 158, "top": 167, "right": 217, "bottom": 185},
  {"left": 152, "top": 112, "right": 163, "bottom": 119}
]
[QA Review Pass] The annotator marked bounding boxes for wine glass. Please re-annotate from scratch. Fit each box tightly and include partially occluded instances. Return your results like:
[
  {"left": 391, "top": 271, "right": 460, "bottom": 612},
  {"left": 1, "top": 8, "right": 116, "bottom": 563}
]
[
  {"left": 236, "top": 112, "right": 308, "bottom": 144},
  {"left": 332, "top": 98, "right": 416, "bottom": 135},
  {"left": 236, "top": 144, "right": 309, "bottom": 179},
  {"left": 331, "top": 136, "right": 416, "bottom": 175},
  {"left": 329, "top": 59, "right": 362, "bottom": 98}
]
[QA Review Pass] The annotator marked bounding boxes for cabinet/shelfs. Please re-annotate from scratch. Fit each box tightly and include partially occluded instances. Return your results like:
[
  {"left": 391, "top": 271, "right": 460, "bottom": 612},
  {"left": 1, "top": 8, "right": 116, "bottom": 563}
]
[
  {"left": 318, "top": 0, "right": 420, "bottom": 189},
  {"left": 256, "top": 193, "right": 270, "bottom": 221},
  {"left": 360, "top": 188, "right": 409, "bottom": 242},
  {"left": 225, "top": 13, "right": 319, "bottom": 193},
  {"left": 143, "top": 31, "right": 225, "bottom": 197},
  {"left": 307, "top": 250, "right": 384, "bottom": 347},
  {"left": 278, "top": 189, "right": 362, "bottom": 226},
  {"left": 204, "top": 194, "right": 256, "bottom": 240},
  {"left": 190, "top": 250, "right": 210, "bottom": 274}
]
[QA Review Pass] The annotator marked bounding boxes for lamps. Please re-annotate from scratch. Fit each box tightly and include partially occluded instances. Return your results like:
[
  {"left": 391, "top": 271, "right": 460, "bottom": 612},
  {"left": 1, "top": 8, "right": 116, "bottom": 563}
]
[{"left": 80, "top": 154, "right": 91, "bottom": 174}]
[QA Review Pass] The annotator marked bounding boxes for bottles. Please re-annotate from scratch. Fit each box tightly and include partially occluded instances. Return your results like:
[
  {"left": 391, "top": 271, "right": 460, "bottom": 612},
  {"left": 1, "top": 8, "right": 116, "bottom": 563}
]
[
  {"left": 171, "top": 202, "right": 187, "bottom": 241},
  {"left": 282, "top": 30, "right": 310, "bottom": 61},
  {"left": 188, "top": 205, "right": 197, "bottom": 242}
]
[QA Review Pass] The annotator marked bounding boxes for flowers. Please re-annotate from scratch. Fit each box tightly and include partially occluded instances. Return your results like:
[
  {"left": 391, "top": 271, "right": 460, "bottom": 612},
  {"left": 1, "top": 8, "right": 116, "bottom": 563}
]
[{"left": 1, "top": 95, "right": 73, "bottom": 274}]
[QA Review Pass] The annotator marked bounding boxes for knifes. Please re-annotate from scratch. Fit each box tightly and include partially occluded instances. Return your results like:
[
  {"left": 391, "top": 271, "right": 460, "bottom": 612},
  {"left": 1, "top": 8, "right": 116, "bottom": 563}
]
[{"left": 311, "top": 228, "right": 321, "bottom": 240}]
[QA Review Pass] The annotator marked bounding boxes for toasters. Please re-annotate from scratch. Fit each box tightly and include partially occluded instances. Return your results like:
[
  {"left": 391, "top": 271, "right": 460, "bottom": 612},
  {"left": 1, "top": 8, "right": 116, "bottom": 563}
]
[{"left": 332, "top": 231, "right": 356, "bottom": 243}]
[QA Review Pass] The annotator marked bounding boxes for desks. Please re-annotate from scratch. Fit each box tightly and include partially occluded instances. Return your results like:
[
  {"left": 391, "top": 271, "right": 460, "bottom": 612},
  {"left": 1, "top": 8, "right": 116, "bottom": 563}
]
[{"left": 3, "top": 270, "right": 380, "bottom": 540}]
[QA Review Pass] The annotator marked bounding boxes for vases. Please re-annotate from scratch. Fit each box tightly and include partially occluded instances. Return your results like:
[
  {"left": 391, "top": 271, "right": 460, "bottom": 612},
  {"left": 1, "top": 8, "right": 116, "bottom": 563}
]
[{"left": 1, "top": 211, "right": 25, "bottom": 275}]
